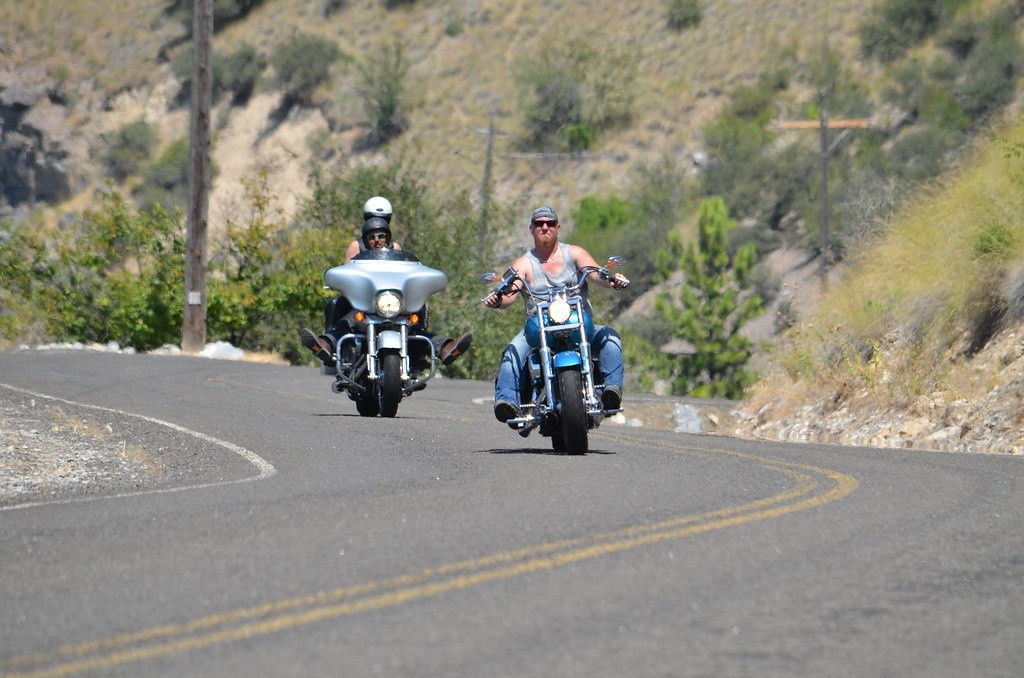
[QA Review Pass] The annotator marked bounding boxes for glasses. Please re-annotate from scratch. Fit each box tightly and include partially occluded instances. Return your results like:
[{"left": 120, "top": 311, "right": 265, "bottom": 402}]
[
  {"left": 531, "top": 220, "right": 557, "bottom": 227},
  {"left": 365, "top": 233, "right": 386, "bottom": 239}
]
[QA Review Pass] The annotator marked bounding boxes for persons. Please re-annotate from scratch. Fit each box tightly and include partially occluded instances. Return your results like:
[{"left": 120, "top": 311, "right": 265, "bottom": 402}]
[
  {"left": 482, "top": 206, "right": 630, "bottom": 429},
  {"left": 297, "top": 196, "right": 474, "bottom": 366}
]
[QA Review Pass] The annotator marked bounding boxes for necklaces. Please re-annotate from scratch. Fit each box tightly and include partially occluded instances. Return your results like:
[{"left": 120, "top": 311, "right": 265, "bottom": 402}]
[{"left": 533, "top": 246, "right": 561, "bottom": 263}]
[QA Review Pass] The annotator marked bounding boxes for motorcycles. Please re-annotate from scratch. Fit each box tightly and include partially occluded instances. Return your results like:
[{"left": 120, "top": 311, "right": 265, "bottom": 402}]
[
  {"left": 480, "top": 256, "right": 629, "bottom": 456},
  {"left": 322, "top": 249, "right": 449, "bottom": 419}
]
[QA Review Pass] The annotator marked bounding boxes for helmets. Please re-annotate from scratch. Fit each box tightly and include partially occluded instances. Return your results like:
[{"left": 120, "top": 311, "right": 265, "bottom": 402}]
[
  {"left": 363, "top": 197, "right": 392, "bottom": 225},
  {"left": 362, "top": 217, "right": 391, "bottom": 249}
]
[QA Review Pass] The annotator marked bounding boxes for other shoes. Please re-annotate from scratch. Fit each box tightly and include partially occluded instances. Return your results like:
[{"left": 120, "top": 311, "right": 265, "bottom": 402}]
[
  {"left": 438, "top": 333, "right": 472, "bottom": 366},
  {"left": 601, "top": 385, "right": 622, "bottom": 418},
  {"left": 493, "top": 400, "right": 525, "bottom": 430},
  {"left": 300, "top": 328, "right": 333, "bottom": 361},
  {"left": 332, "top": 373, "right": 349, "bottom": 393},
  {"left": 402, "top": 377, "right": 426, "bottom": 392}
]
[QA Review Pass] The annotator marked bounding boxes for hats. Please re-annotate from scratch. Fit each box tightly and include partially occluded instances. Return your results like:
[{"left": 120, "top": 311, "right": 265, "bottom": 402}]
[{"left": 531, "top": 206, "right": 557, "bottom": 221}]
